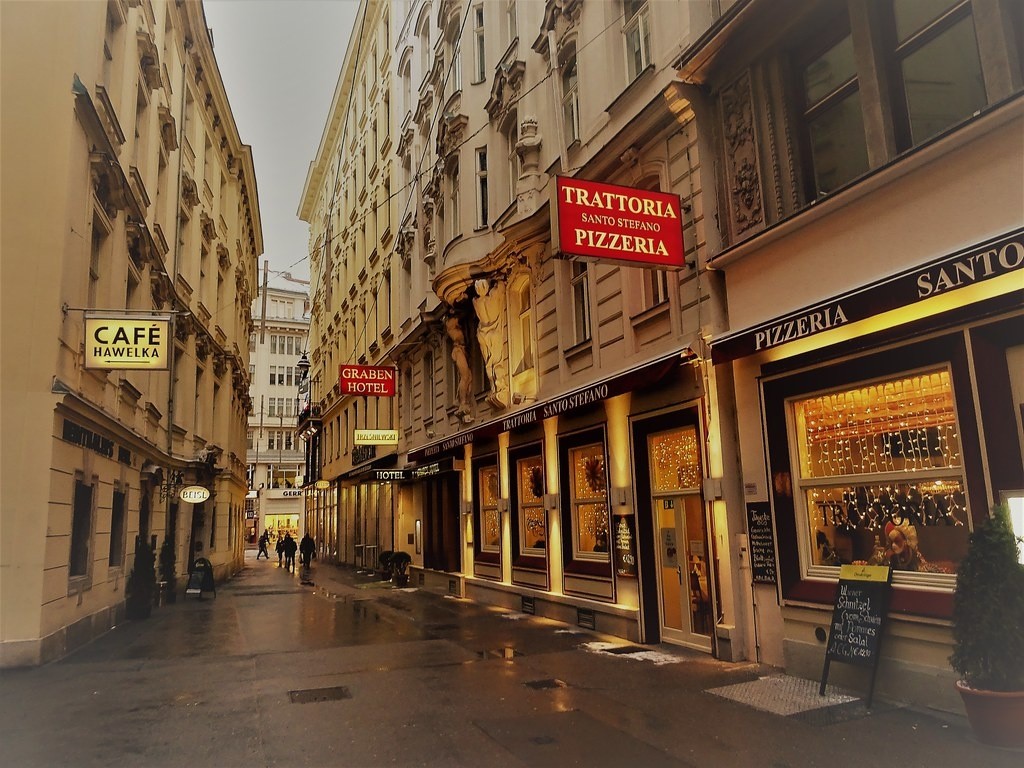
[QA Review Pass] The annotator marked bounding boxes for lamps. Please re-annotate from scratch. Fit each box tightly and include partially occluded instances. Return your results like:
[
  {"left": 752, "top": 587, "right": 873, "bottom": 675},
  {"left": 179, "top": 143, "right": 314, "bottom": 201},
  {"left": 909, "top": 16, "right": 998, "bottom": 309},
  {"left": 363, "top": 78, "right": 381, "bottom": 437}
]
[{"left": 297, "top": 348, "right": 312, "bottom": 371}]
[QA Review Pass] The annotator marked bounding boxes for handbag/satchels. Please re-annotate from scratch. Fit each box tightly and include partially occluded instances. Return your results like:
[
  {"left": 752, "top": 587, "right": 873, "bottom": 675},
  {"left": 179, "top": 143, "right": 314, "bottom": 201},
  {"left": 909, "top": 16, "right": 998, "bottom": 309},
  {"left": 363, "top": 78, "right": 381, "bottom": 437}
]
[
  {"left": 312, "top": 552, "right": 317, "bottom": 559},
  {"left": 298, "top": 552, "right": 303, "bottom": 564}
]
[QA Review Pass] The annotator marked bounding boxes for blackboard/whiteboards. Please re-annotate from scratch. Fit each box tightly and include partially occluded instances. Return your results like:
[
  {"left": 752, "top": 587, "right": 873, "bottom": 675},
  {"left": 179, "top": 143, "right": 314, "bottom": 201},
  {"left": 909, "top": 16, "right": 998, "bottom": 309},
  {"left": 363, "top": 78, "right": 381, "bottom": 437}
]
[{"left": 827, "top": 580, "right": 890, "bottom": 670}]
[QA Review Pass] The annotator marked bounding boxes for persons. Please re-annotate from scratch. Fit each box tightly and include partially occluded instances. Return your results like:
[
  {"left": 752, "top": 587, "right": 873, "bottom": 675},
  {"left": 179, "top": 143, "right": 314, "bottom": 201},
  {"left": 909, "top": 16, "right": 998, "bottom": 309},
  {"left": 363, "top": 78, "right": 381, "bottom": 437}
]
[
  {"left": 445, "top": 306, "right": 474, "bottom": 415},
  {"left": 263, "top": 529, "right": 272, "bottom": 545},
  {"left": 257, "top": 535, "right": 270, "bottom": 560},
  {"left": 275, "top": 533, "right": 297, "bottom": 574},
  {"left": 300, "top": 533, "right": 315, "bottom": 568},
  {"left": 868, "top": 517, "right": 936, "bottom": 572},
  {"left": 815, "top": 530, "right": 851, "bottom": 565},
  {"left": 472, "top": 280, "right": 509, "bottom": 391}
]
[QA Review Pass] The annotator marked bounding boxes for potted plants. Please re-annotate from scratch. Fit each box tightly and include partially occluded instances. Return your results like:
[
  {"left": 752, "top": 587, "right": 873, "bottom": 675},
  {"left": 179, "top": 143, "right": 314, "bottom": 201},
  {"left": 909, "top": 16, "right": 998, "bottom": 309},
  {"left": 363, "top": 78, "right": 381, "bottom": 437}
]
[
  {"left": 948, "top": 503, "right": 1024, "bottom": 745},
  {"left": 379, "top": 551, "right": 394, "bottom": 581},
  {"left": 389, "top": 552, "right": 411, "bottom": 587}
]
